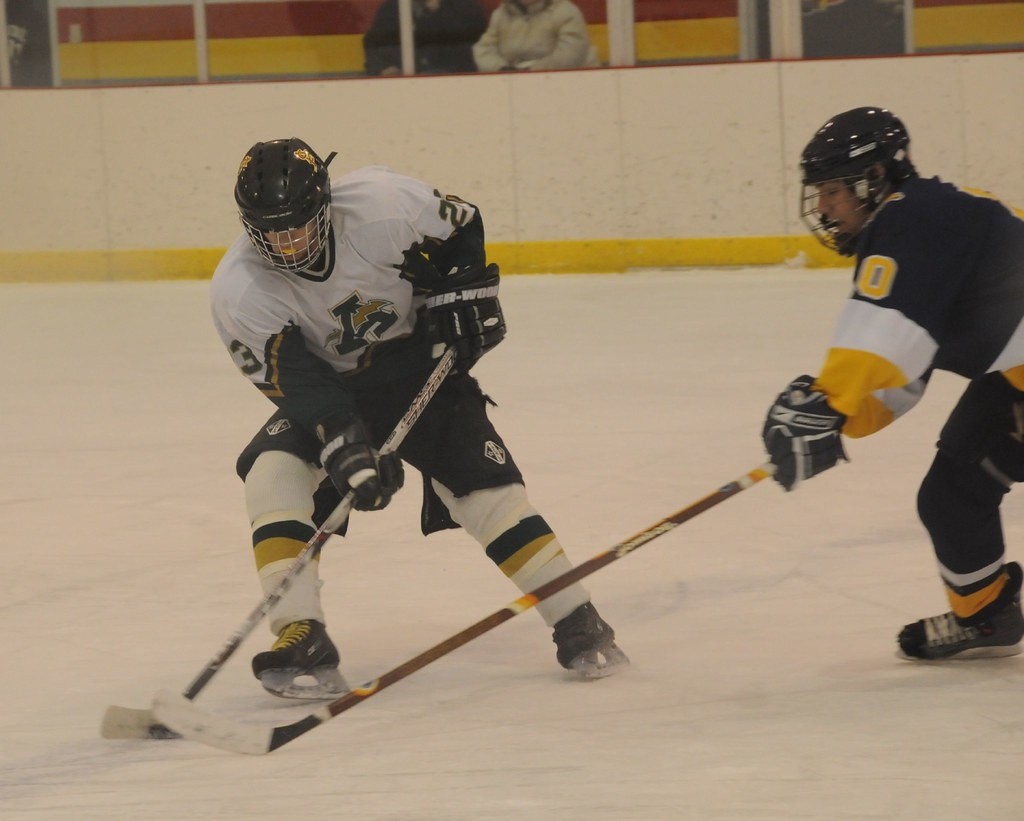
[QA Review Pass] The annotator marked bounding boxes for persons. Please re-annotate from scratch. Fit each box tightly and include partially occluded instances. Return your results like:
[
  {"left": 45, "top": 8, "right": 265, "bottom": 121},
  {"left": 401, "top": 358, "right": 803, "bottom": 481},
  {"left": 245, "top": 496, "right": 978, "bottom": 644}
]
[
  {"left": 763, "top": 106, "right": 1023, "bottom": 659},
  {"left": 209, "top": 138, "right": 628, "bottom": 698},
  {"left": 470, "top": 0, "right": 600, "bottom": 72},
  {"left": 5, "top": 0, "right": 51, "bottom": 85},
  {"left": 364, "top": 0, "right": 482, "bottom": 77}
]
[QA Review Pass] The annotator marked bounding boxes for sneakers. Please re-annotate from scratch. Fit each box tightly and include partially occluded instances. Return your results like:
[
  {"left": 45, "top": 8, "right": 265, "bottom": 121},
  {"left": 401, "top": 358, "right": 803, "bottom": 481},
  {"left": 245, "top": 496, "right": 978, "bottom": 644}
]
[
  {"left": 554, "top": 604, "right": 628, "bottom": 681},
  {"left": 898, "top": 563, "right": 1024, "bottom": 661},
  {"left": 253, "top": 618, "right": 349, "bottom": 701}
]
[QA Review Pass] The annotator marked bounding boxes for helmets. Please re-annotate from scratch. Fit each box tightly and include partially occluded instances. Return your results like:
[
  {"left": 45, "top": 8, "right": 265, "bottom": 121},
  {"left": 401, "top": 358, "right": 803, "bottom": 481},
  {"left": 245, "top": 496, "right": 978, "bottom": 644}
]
[
  {"left": 799, "top": 108, "right": 914, "bottom": 256},
  {"left": 234, "top": 139, "right": 337, "bottom": 273}
]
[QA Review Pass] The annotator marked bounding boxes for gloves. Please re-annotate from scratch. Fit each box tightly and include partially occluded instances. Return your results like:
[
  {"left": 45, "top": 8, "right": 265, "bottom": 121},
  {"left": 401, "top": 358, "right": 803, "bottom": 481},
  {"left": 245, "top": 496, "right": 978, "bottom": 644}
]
[
  {"left": 319, "top": 418, "right": 404, "bottom": 512},
  {"left": 763, "top": 375, "right": 849, "bottom": 491},
  {"left": 420, "top": 263, "right": 507, "bottom": 382}
]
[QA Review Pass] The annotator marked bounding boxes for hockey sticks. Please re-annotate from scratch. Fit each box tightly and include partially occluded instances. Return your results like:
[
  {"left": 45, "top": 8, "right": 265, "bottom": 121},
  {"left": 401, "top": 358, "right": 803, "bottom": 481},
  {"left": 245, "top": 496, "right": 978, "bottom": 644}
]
[
  {"left": 152, "top": 458, "right": 778, "bottom": 755},
  {"left": 100, "top": 339, "right": 459, "bottom": 741}
]
